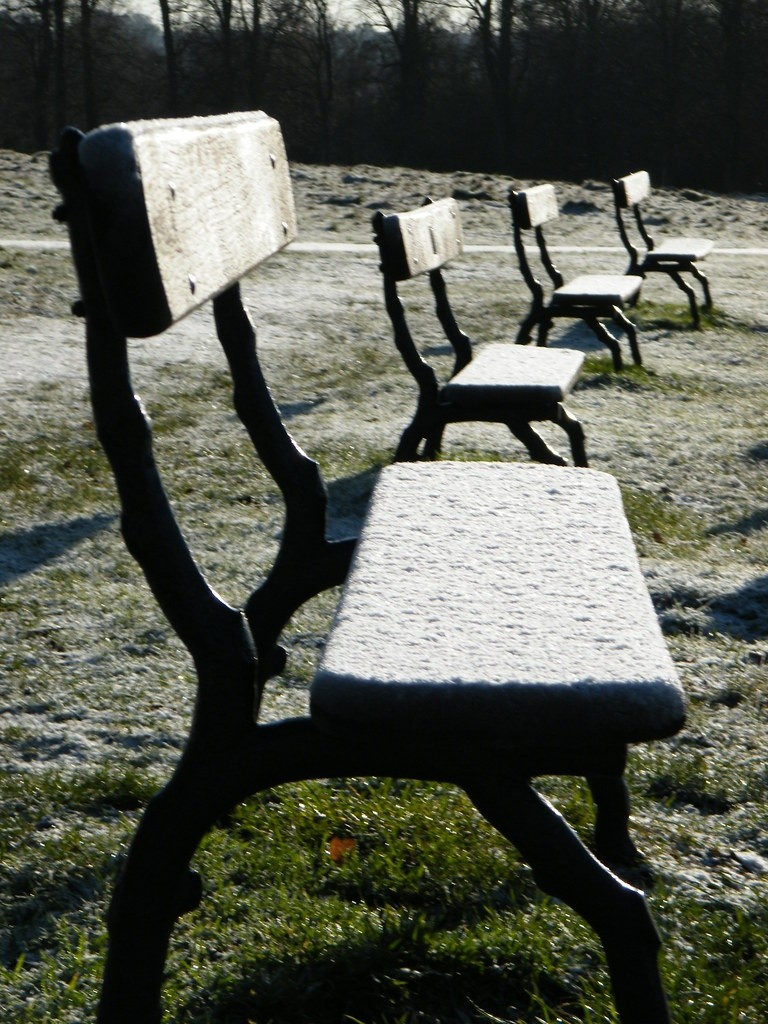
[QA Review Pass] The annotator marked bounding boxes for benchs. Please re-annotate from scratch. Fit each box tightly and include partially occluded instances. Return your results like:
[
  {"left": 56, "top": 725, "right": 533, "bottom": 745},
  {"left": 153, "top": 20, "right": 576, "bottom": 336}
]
[
  {"left": 368, "top": 195, "right": 590, "bottom": 470},
  {"left": 48, "top": 109, "right": 687, "bottom": 1024},
  {"left": 508, "top": 184, "right": 646, "bottom": 376},
  {"left": 611, "top": 171, "right": 713, "bottom": 331}
]
